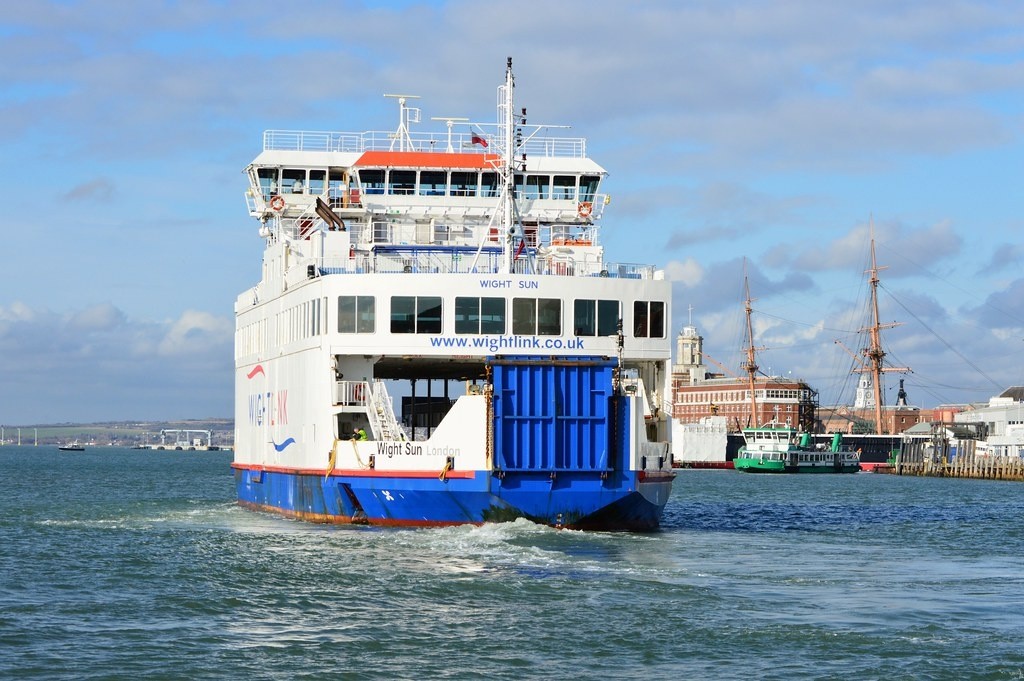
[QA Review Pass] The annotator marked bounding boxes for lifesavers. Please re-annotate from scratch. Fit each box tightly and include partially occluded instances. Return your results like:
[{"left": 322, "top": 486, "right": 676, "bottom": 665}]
[
  {"left": 577, "top": 202, "right": 592, "bottom": 217},
  {"left": 270, "top": 195, "right": 285, "bottom": 211},
  {"left": 353, "top": 383, "right": 366, "bottom": 401}
]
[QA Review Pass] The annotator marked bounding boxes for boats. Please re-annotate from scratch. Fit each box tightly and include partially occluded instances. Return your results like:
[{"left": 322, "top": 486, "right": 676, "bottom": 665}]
[
  {"left": 229, "top": 56, "right": 678, "bottom": 533},
  {"left": 732, "top": 420, "right": 864, "bottom": 474},
  {"left": 59, "top": 443, "right": 85, "bottom": 450}
]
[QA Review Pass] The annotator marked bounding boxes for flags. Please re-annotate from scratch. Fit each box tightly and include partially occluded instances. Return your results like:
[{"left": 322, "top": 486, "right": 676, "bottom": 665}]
[{"left": 471, "top": 131, "right": 488, "bottom": 147}]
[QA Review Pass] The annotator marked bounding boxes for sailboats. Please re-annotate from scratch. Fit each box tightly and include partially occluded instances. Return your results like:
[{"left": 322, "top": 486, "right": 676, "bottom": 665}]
[{"left": 692, "top": 213, "right": 1024, "bottom": 473}]
